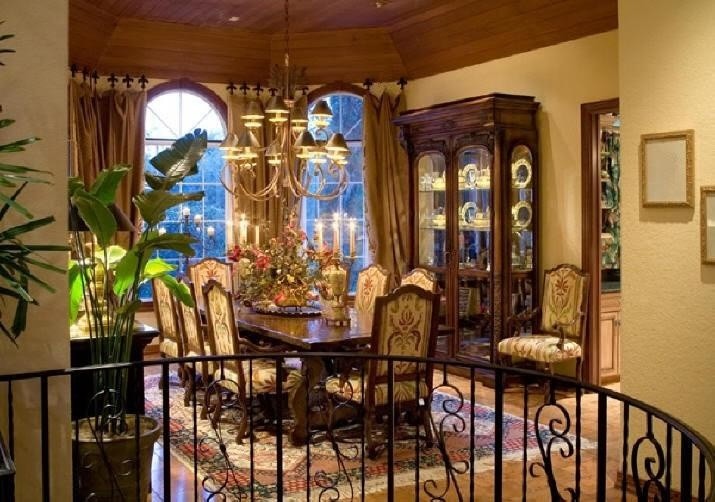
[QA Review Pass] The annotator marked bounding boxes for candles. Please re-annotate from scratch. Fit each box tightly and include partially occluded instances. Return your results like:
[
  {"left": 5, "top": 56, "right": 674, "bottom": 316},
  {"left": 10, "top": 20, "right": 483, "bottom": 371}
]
[
  {"left": 255, "top": 226, "right": 260, "bottom": 244},
  {"left": 240, "top": 222, "right": 244, "bottom": 243},
  {"left": 245, "top": 221, "right": 248, "bottom": 242},
  {"left": 207, "top": 226, "right": 215, "bottom": 239},
  {"left": 332, "top": 222, "right": 340, "bottom": 248},
  {"left": 350, "top": 222, "right": 356, "bottom": 257},
  {"left": 316, "top": 220, "right": 325, "bottom": 249}
]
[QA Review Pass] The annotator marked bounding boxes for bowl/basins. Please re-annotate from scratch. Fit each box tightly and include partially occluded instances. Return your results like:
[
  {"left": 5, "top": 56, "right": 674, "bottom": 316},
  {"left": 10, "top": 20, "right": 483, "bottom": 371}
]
[
  {"left": 512, "top": 226, "right": 522, "bottom": 232},
  {"left": 431, "top": 183, "right": 445, "bottom": 189},
  {"left": 474, "top": 219, "right": 489, "bottom": 226}
]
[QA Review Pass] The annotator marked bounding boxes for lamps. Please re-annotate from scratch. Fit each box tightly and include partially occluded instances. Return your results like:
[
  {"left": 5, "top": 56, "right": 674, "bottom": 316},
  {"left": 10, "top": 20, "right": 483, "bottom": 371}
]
[{"left": 218, "top": 0, "right": 351, "bottom": 202}]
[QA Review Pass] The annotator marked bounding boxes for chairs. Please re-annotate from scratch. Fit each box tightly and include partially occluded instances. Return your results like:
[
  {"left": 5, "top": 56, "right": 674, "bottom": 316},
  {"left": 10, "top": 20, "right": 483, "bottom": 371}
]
[{"left": 496, "top": 263, "right": 591, "bottom": 404}]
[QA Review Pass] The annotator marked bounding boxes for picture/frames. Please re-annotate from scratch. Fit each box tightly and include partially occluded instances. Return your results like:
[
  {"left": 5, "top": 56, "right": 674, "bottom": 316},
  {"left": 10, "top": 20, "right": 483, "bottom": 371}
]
[
  {"left": 640, "top": 129, "right": 696, "bottom": 209},
  {"left": 699, "top": 184, "right": 715, "bottom": 264}
]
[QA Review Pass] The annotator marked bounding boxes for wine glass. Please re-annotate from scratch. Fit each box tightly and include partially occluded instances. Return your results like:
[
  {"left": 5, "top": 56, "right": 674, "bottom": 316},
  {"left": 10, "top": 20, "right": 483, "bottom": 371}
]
[{"left": 468, "top": 169, "right": 476, "bottom": 190}]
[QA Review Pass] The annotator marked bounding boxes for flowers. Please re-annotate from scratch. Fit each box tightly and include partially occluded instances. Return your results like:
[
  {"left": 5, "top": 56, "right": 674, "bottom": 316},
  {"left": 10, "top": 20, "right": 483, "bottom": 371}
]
[{"left": 226, "top": 219, "right": 333, "bottom": 306}]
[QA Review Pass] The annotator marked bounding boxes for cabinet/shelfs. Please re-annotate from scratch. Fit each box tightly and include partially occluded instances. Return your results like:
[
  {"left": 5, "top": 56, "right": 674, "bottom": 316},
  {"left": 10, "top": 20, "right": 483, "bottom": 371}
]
[
  {"left": 600, "top": 292, "right": 620, "bottom": 386},
  {"left": 600, "top": 126, "right": 620, "bottom": 270}
]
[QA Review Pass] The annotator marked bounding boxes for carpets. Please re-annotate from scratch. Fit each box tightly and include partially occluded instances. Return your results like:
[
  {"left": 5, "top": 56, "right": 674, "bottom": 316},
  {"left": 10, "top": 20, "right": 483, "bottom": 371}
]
[{"left": 144, "top": 363, "right": 598, "bottom": 502}]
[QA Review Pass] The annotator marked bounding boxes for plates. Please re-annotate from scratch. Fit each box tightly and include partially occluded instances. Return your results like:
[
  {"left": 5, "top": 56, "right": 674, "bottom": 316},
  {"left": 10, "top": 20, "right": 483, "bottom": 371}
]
[
  {"left": 462, "top": 163, "right": 477, "bottom": 177},
  {"left": 461, "top": 202, "right": 477, "bottom": 224},
  {"left": 468, "top": 224, "right": 490, "bottom": 229},
  {"left": 513, "top": 158, "right": 532, "bottom": 188},
  {"left": 513, "top": 201, "right": 532, "bottom": 230}
]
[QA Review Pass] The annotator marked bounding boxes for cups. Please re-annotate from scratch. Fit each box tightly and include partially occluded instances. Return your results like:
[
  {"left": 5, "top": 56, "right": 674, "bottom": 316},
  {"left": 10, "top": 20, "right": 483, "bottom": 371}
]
[{"left": 419, "top": 172, "right": 432, "bottom": 191}]
